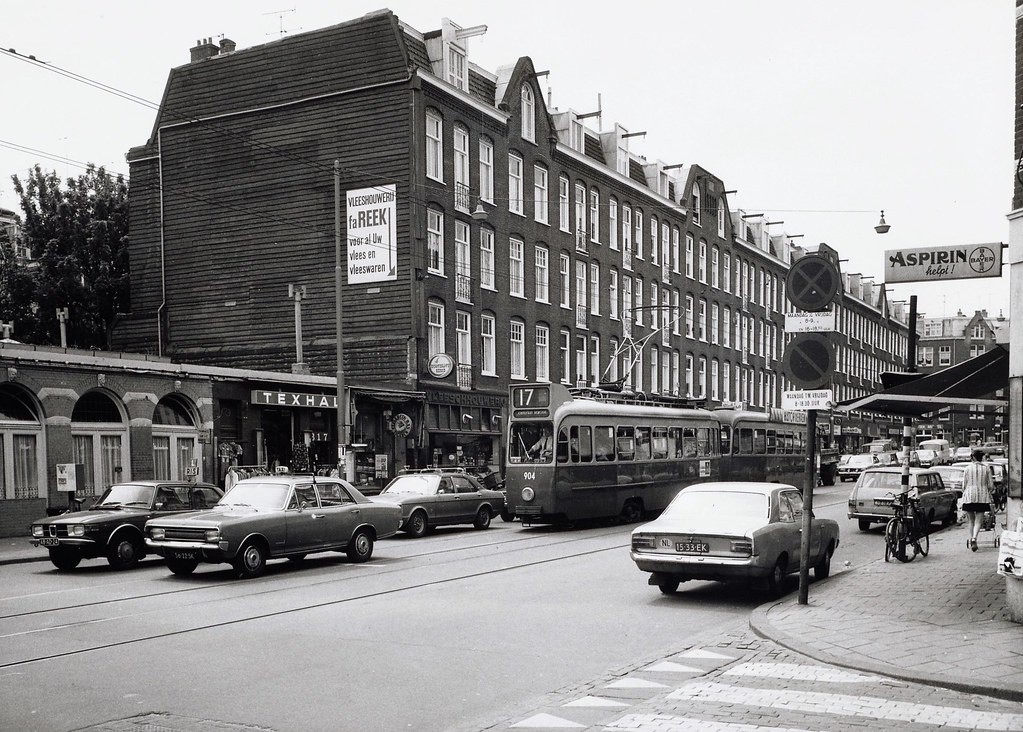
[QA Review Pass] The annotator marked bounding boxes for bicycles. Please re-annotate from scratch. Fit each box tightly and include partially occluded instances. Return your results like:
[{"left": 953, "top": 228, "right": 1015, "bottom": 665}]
[{"left": 879, "top": 485, "right": 931, "bottom": 563}]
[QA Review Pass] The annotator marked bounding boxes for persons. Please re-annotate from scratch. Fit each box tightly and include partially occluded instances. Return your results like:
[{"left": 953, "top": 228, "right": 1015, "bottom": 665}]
[
  {"left": 961, "top": 450, "right": 994, "bottom": 552},
  {"left": 440, "top": 480, "right": 452, "bottom": 493},
  {"left": 526, "top": 426, "right": 553, "bottom": 457}
]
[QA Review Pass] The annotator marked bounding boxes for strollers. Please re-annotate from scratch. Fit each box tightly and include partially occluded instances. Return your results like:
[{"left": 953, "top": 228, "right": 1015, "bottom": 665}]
[{"left": 981, "top": 488, "right": 997, "bottom": 531}]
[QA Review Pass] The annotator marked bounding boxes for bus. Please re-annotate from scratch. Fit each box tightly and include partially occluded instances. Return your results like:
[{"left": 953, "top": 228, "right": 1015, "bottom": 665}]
[{"left": 502, "top": 304, "right": 823, "bottom": 531}]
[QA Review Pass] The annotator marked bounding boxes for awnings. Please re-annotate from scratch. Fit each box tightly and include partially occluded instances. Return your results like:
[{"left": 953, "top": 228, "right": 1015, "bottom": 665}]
[{"left": 827, "top": 346, "right": 1009, "bottom": 418}]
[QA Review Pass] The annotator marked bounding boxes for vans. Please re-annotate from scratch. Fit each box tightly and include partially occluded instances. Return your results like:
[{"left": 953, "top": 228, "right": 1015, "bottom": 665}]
[
  {"left": 858, "top": 439, "right": 897, "bottom": 457},
  {"left": 920, "top": 438, "right": 951, "bottom": 465}
]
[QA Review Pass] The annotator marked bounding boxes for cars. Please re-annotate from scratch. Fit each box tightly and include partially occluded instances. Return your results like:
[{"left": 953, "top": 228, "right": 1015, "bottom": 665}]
[
  {"left": 928, "top": 458, "right": 1009, "bottom": 498},
  {"left": 847, "top": 468, "right": 958, "bottom": 529},
  {"left": 972, "top": 441, "right": 1005, "bottom": 455},
  {"left": 630, "top": 482, "right": 841, "bottom": 597},
  {"left": 26, "top": 480, "right": 225, "bottom": 569},
  {"left": 143, "top": 471, "right": 404, "bottom": 579},
  {"left": 376, "top": 470, "right": 504, "bottom": 538},
  {"left": 948, "top": 446, "right": 974, "bottom": 463},
  {"left": 837, "top": 449, "right": 939, "bottom": 482}
]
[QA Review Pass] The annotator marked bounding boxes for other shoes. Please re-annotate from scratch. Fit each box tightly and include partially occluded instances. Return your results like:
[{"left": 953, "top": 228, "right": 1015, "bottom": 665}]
[{"left": 970, "top": 538, "right": 978, "bottom": 551}]
